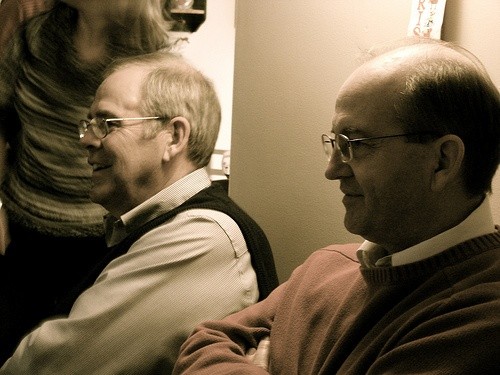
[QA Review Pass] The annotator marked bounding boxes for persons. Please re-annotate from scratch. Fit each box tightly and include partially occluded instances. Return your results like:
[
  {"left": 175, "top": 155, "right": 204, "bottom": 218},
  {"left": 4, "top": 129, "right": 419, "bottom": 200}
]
[
  {"left": 0, "top": 0, "right": 172, "bottom": 367},
  {"left": 173, "top": 38, "right": 500, "bottom": 375},
  {"left": 1, "top": 53, "right": 279, "bottom": 375}
]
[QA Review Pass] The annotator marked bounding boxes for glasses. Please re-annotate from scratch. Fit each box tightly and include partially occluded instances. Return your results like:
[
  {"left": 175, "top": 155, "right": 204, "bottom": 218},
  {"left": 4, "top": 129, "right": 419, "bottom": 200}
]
[
  {"left": 320, "top": 133, "right": 406, "bottom": 164},
  {"left": 78, "top": 117, "right": 169, "bottom": 140}
]
